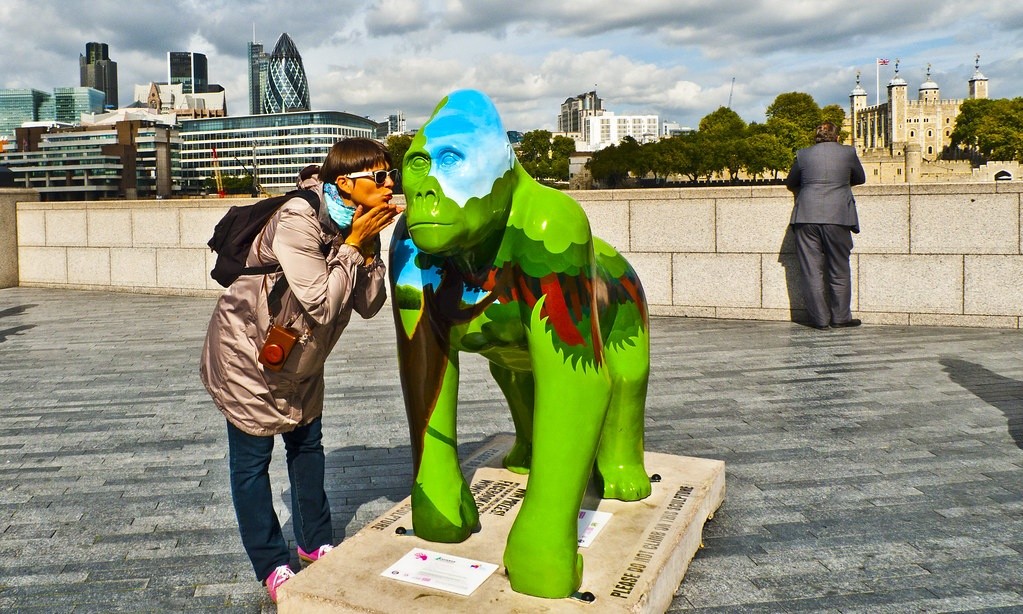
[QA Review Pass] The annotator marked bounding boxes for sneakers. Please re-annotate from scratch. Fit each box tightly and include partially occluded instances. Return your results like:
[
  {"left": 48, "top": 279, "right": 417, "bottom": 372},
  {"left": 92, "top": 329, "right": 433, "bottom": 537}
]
[
  {"left": 265, "top": 564, "right": 295, "bottom": 603},
  {"left": 297, "top": 544, "right": 334, "bottom": 562}
]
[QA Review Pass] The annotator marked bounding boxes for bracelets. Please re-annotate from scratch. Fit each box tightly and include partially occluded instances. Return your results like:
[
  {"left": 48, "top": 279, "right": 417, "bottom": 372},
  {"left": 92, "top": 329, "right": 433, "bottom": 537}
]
[{"left": 347, "top": 242, "right": 360, "bottom": 249}]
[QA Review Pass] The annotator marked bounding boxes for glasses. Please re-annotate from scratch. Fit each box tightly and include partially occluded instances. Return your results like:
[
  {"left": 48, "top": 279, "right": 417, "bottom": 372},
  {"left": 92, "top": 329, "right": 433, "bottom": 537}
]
[{"left": 334, "top": 168, "right": 398, "bottom": 185}]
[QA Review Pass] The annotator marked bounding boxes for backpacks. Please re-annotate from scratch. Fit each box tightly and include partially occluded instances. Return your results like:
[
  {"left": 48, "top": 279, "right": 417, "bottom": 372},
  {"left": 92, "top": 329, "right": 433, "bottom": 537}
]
[{"left": 207, "top": 189, "right": 331, "bottom": 288}]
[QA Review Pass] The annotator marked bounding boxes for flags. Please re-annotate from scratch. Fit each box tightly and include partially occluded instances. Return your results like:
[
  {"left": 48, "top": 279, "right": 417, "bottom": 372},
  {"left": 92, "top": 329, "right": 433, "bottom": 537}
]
[{"left": 878, "top": 59, "right": 889, "bottom": 65}]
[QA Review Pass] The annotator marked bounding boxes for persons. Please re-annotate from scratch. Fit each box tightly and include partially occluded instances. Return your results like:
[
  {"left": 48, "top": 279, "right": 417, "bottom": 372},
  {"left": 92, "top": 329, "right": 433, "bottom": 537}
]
[
  {"left": 200, "top": 136, "right": 405, "bottom": 605},
  {"left": 786, "top": 123, "right": 865, "bottom": 329}
]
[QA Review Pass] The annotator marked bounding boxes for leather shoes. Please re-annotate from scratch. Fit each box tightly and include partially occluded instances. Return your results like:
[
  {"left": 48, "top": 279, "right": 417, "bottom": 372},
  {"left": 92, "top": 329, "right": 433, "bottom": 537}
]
[
  {"left": 832, "top": 319, "right": 861, "bottom": 328},
  {"left": 816, "top": 325, "right": 829, "bottom": 330}
]
